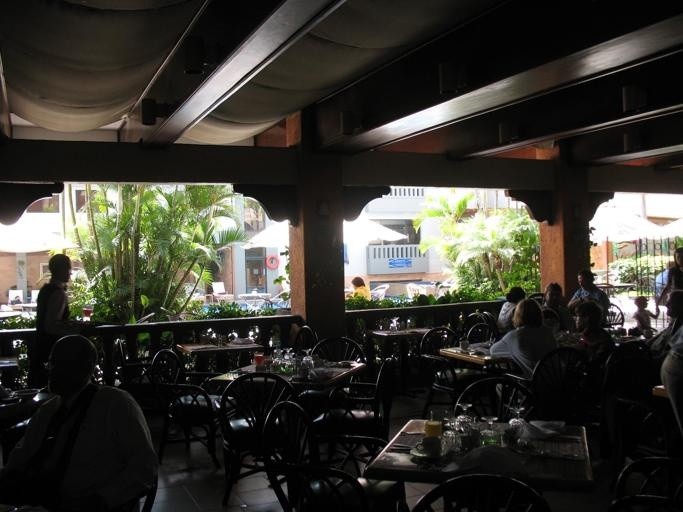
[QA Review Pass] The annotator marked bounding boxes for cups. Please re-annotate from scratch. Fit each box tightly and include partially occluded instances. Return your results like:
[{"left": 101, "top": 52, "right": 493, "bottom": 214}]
[
  {"left": 416, "top": 419, "right": 461, "bottom": 456},
  {"left": 253, "top": 350, "right": 265, "bottom": 365},
  {"left": 459, "top": 337, "right": 469, "bottom": 350}
]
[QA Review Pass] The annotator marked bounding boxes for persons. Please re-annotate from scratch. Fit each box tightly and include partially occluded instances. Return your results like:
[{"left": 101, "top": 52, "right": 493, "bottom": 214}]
[
  {"left": 10, "top": 295, "right": 22, "bottom": 306},
  {"left": 488, "top": 299, "right": 554, "bottom": 402},
  {"left": 496, "top": 286, "right": 526, "bottom": 337},
  {"left": 542, "top": 281, "right": 576, "bottom": 335},
  {"left": 23, "top": 253, "right": 72, "bottom": 392},
  {"left": 565, "top": 271, "right": 612, "bottom": 311},
  {"left": 0, "top": 334, "right": 161, "bottom": 512},
  {"left": 555, "top": 301, "right": 633, "bottom": 395},
  {"left": 629, "top": 245, "right": 683, "bottom": 436},
  {"left": 350, "top": 276, "right": 371, "bottom": 302}
]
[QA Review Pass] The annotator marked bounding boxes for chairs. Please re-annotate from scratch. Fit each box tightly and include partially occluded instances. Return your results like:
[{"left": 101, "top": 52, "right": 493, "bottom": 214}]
[
  {"left": 607, "top": 456, "right": 683, "bottom": 512},
  {"left": 349, "top": 282, "right": 683, "bottom": 415},
  {"left": 115, "top": 326, "right": 406, "bottom": 511},
  {"left": 412, "top": 473, "right": 547, "bottom": 512},
  {"left": 1, "top": 303, "right": 49, "bottom": 463},
  {"left": 184, "top": 283, "right": 290, "bottom": 305}
]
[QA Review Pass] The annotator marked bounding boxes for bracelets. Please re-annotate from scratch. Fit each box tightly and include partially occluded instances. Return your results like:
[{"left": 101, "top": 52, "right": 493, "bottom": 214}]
[{"left": 578, "top": 296, "right": 583, "bottom": 303}]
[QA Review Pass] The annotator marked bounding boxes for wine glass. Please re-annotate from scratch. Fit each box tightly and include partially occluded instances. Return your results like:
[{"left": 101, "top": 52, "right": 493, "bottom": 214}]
[
  {"left": 456, "top": 401, "right": 527, "bottom": 447},
  {"left": 271, "top": 346, "right": 314, "bottom": 375},
  {"left": 389, "top": 317, "right": 402, "bottom": 333}
]
